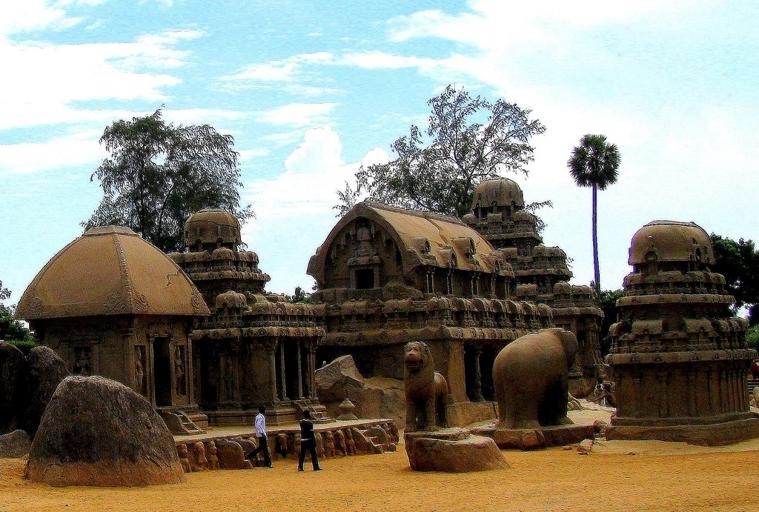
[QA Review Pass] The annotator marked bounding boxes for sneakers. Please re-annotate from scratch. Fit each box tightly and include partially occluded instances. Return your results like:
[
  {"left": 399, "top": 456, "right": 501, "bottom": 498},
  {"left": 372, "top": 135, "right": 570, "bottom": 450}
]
[{"left": 246, "top": 453, "right": 323, "bottom": 472}]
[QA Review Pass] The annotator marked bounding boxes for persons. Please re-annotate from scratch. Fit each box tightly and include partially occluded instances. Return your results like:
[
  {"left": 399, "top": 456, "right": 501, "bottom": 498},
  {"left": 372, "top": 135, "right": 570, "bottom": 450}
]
[
  {"left": 175, "top": 346, "right": 184, "bottom": 395},
  {"left": 245, "top": 404, "right": 272, "bottom": 467},
  {"left": 135, "top": 347, "right": 143, "bottom": 393},
  {"left": 298, "top": 409, "right": 323, "bottom": 471}
]
